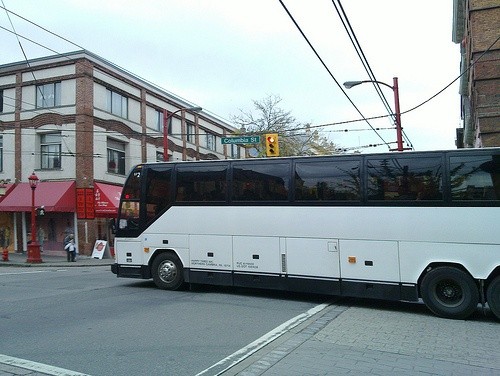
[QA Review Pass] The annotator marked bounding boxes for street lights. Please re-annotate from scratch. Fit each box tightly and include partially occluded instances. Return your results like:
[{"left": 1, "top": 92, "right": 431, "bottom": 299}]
[
  {"left": 24, "top": 171, "right": 44, "bottom": 263},
  {"left": 342, "top": 77, "right": 404, "bottom": 151},
  {"left": 163, "top": 105, "right": 202, "bottom": 162}
]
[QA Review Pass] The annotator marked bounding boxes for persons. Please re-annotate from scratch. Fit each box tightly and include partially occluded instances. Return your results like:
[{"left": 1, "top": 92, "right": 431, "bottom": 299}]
[{"left": 64, "top": 234, "right": 77, "bottom": 262}]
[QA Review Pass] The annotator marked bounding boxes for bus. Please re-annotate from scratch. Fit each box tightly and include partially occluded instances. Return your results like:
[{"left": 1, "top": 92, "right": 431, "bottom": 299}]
[{"left": 110, "top": 147, "right": 500, "bottom": 323}]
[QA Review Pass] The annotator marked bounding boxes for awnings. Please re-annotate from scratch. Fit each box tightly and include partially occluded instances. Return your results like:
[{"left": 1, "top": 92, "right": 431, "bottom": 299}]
[
  {"left": 94, "top": 183, "right": 156, "bottom": 217},
  {"left": 0, "top": 181, "right": 76, "bottom": 211}
]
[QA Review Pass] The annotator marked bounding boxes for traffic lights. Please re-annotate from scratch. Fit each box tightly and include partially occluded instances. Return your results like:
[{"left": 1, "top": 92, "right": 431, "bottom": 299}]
[{"left": 265, "top": 133, "right": 279, "bottom": 157}]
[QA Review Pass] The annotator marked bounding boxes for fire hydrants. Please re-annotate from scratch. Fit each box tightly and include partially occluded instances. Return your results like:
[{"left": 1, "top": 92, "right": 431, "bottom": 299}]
[{"left": 1, "top": 246, "right": 9, "bottom": 262}]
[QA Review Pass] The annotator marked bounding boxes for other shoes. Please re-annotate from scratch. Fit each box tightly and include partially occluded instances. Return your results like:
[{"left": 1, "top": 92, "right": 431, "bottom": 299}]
[{"left": 68, "top": 260, "right": 76, "bottom": 262}]
